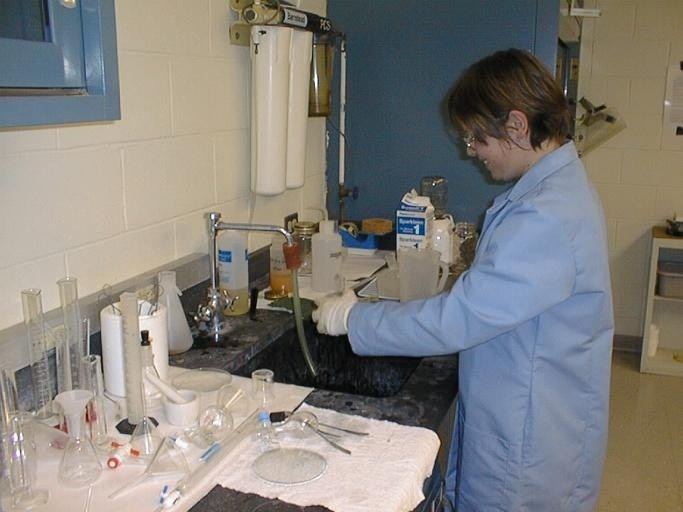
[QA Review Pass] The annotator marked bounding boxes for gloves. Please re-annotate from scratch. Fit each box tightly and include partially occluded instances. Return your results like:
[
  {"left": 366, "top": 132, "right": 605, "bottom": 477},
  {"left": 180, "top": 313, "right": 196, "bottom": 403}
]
[{"left": 311, "top": 287, "right": 358, "bottom": 336}]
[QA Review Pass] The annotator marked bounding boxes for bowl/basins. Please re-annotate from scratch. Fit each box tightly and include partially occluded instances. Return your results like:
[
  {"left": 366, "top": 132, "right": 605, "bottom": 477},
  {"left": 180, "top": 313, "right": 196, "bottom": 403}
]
[{"left": 659, "top": 260, "right": 683, "bottom": 299}]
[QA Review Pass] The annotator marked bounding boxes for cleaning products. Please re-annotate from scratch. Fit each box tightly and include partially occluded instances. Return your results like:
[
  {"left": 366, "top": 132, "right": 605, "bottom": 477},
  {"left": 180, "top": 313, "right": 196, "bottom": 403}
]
[{"left": 268, "top": 233, "right": 292, "bottom": 293}]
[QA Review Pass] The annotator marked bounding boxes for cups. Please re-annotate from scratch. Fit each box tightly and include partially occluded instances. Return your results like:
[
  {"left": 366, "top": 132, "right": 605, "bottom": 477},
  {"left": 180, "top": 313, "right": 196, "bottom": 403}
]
[
  {"left": 100, "top": 301, "right": 172, "bottom": 396},
  {"left": 162, "top": 388, "right": 200, "bottom": 426},
  {"left": 378, "top": 272, "right": 400, "bottom": 298}
]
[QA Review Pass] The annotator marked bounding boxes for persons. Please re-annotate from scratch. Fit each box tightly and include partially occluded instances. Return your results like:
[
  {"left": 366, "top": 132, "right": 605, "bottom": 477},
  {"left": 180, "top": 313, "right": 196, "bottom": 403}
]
[{"left": 311, "top": 48, "right": 615, "bottom": 512}]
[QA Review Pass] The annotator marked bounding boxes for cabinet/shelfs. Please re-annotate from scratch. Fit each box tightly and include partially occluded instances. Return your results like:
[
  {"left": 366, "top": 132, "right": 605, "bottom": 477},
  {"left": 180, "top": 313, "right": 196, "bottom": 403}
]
[{"left": 638, "top": 225, "right": 682, "bottom": 377}]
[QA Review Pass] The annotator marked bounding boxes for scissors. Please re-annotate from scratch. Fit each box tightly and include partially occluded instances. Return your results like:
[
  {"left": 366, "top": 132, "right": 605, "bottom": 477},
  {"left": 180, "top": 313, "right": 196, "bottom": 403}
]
[{"left": 99, "top": 283, "right": 164, "bottom": 316}]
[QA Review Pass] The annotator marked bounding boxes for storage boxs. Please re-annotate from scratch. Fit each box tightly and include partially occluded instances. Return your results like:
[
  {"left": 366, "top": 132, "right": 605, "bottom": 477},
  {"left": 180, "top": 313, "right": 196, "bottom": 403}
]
[{"left": 655, "top": 259, "right": 683, "bottom": 300}]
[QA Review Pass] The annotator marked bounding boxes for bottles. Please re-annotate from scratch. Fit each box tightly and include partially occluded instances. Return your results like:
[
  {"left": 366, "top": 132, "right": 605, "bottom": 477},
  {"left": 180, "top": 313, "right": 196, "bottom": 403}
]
[
  {"left": 118, "top": 289, "right": 157, "bottom": 435},
  {"left": 191, "top": 366, "right": 318, "bottom": 461},
  {"left": 307, "top": 30, "right": 333, "bottom": 120},
  {"left": 156, "top": 268, "right": 195, "bottom": 354},
  {"left": 1, "top": 278, "right": 103, "bottom": 510},
  {"left": 292, "top": 221, "right": 316, "bottom": 276},
  {"left": 451, "top": 222, "right": 479, "bottom": 272},
  {"left": 648, "top": 324, "right": 661, "bottom": 359},
  {"left": 268, "top": 232, "right": 296, "bottom": 294}
]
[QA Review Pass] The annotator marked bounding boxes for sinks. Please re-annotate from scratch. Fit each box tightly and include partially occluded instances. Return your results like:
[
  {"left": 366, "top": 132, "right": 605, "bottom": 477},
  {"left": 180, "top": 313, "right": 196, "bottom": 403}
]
[{"left": 221, "top": 304, "right": 438, "bottom": 397}]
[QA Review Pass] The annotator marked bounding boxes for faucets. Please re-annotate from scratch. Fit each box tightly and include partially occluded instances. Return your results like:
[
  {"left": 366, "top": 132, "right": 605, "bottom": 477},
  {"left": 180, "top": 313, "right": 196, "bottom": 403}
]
[{"left": 217, "top": 223, "right": 302, "bottom": 269}]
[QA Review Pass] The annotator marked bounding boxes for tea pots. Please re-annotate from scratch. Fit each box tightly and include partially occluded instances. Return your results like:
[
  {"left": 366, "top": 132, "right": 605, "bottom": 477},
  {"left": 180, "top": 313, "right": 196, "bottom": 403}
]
[{"left": 385, "top": 245, "right": 448, "bottom": 305}]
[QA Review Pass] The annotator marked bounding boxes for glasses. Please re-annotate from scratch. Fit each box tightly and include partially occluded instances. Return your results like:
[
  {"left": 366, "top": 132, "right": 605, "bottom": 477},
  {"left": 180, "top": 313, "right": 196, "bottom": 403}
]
[{"left": 462, "top": 125, "right": 492, "bottom": 148}]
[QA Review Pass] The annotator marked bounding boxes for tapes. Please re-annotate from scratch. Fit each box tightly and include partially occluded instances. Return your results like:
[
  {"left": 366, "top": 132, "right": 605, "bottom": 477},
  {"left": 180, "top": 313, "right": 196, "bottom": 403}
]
[{"left": 340, "top": 222, "right": 359, "bottom": 238}]
